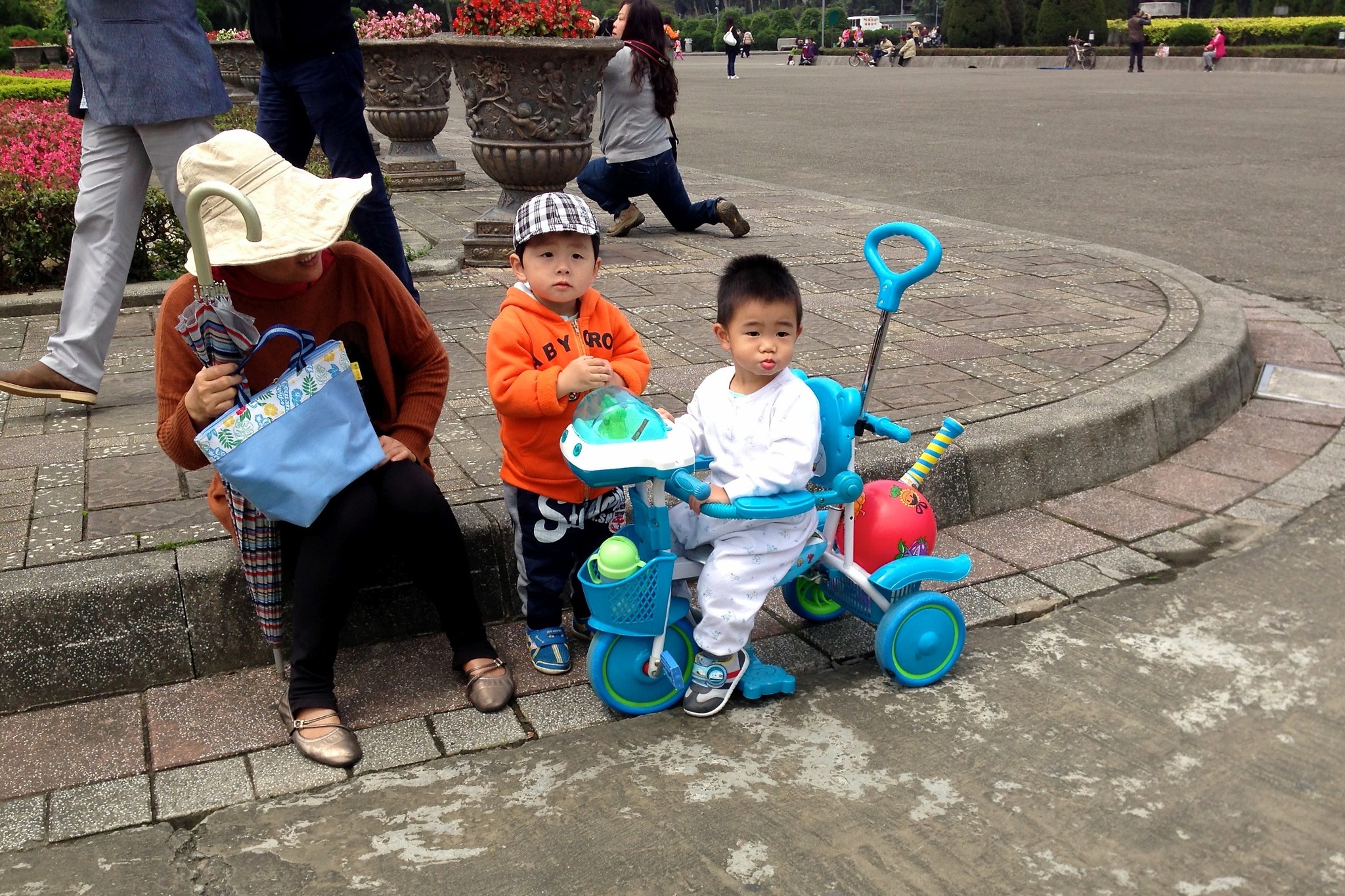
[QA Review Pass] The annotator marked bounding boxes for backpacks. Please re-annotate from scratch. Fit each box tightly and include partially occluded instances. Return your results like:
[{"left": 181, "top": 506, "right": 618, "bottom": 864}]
[{"left": 674, "top": 41, "right": 678, "bottom": 48}]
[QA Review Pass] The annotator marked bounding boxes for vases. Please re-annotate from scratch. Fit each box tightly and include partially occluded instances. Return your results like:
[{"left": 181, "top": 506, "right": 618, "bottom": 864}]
[
  {"left": 209, "top": 41, "right": 264, "bottom": 106},
  {"left": 358, "top": 38, "right": 467, "bottom": 192},
  {"left": 425, "top": 33, "right": 624, "bottom": 268}
]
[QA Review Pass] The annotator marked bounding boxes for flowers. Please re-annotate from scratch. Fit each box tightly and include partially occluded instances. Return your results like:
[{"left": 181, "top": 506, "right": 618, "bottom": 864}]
[
  {"left": 453, "top": 0, "right": 596, "bottom": 39},
  {"left": 354, "top": 3, "right": 443, "bottom": 39},
  {"left": 206, "top": 28, "right": 252, "bottom": 41}
]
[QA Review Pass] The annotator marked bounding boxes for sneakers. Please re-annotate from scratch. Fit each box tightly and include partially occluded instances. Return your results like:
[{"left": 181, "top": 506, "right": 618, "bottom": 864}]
[
  {"left": 869, "top": 60, "right": 876, "bottom": 63},
  {"left": 606, "top": 202, "right": 645, "bottom": 237},
  {"left": 1208, "top": 68, "right": 1213, "bottom": 72},
  {"left": 869, "top": 65, "right": 875, "bottom": 67},
  {"left": 730, "top": 75, "right": 739, "bottom": 79},
  {"left": 1204, "top": 66, "right": 1208, "bottom": 72},
  {"left": 885, "top": 53, "right": 889, "bottom": 57},
  {"left": 572, "top": 616, "right": 597, "bottom": 641},
  {"left": 525, "top": 626, "right": 571, "bottom": 674},
  {"left": 683, "top": 647, "right": 750, "bottom": 717},
  {"left": 727, "top": 76, "right": 730, "bottom": 79},
  {"left": 717, "top": 200, "right": 750, "bottom": 238}
]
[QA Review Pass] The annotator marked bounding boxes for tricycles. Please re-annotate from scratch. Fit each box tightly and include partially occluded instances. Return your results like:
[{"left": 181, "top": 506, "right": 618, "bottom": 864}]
[{"left": 558, "top": 218, "right": 974, "bottom": 714}]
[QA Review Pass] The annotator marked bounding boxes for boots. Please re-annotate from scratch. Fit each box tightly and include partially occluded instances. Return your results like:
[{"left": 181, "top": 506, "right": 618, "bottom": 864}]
[
  {"left": 1128, "top": 66, "right": 1133, "bottom": 72},
  {"left": 1138, "top": 66, "right": 1144, "bottom": 72}
]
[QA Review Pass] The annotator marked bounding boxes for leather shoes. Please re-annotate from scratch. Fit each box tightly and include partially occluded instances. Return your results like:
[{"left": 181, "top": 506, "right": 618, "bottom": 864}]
[{"left": 0, "top": 361, "right": 98, "bottom": 405}]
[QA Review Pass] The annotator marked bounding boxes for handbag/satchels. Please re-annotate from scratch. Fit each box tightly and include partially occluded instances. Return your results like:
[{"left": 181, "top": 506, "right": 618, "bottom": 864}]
[
  {"left": 664, "top": 26, "right": 676, "bottom": 47},
  {"left": 1154, "top": 43, "right": 1170, "bottom": 57},
  {"left": 723, "top": 26, "right": 737, "bottom": 46},
  {"left": 193, "top": 324, "right": 387, "bottom": 528}
]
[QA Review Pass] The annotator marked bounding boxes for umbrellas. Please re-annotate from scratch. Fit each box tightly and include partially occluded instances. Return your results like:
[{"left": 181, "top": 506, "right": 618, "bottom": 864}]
[
  {"left": 910, "top": 21, "right": 921, "bottom": 26},
  {"left": 172, "top": 178, "right": 287, "bottom": 674}
]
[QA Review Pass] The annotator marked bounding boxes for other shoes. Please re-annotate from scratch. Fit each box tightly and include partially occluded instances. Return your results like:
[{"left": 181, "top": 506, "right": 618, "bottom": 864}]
[
  {"left": 897, "top": 65, "right": 903, "bottom": 68},
  {"left": 463, "top": 655, "right": 514, "bottom": 712},
  {"left": 278, "top": 686, "right": 362, "bottom": 767}
]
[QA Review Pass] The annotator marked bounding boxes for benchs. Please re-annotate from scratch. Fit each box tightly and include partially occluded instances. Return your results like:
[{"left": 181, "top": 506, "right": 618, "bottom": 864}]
[{"left": 777, "top": 38, "right": 798, "bottom": 51}]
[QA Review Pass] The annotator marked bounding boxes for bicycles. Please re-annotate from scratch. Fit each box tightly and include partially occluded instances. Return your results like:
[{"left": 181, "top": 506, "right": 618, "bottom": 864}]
[
  {"left": 1064, "top": 36, "right": 1097, "bottom": 70},
  {"left": 848, "top": 47, "right": 874, "bottom": 67}
]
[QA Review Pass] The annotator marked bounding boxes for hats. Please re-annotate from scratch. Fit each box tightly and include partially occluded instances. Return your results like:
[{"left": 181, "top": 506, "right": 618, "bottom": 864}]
[
  {"left": 176, "top": 129, "right": 373, "bottom": 277},
  {"left": 900, "top": 35, "right": 905, "bottom": 38},
  {"left": 513, "top": 192, "right": 600, "bottom": 255}
]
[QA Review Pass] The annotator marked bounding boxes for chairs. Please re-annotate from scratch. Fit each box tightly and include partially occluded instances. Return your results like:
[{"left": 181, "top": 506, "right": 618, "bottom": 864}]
[{"left": 683, "top": 367, "right": 860, "bottom": 590}]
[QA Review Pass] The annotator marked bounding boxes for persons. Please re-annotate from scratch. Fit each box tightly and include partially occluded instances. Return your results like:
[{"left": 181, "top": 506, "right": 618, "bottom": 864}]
[
  {"left": 673, "top": 37, "right": 685, "bottom": 61},
  {"left": 576, "top": 2, "right": 752, "bottom": 242},
  {"left": 661, "top": 13, "right": 680, "bottom": 69},
  {"left": 1126, "top": 8, "right": 1152, "bottom": 74},
  {"left": 155, "top": 127, "right": 517, "bottom": 768},
  {"left": 486, "top": 192, "right": 652, "bottom": 676},
  {"left": 724, "top": 17, "right": 741, "bottom": 79},
  {"left": 244, "top": 0, "right": 422, "bottom": 305},
  {"left": 735, "top": 25, "right": 950, "bottom": 70},
  {"left": 662, "top": 253, "right": 825, "bottom": 719},
  {"left": 596, "top": 12, "right": 618, "bottom": 38},
  {"left": 0, "top": 0, "right": 234, "bottom": 410},
  {"left": 1203, "top": 26, "right": 1226, "bottom": 74}
]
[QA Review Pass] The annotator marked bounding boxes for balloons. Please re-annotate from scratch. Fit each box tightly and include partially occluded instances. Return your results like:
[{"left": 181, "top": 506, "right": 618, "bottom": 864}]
[{"left": 834, "top": 480, "right": 937, "bottom": 571}]
